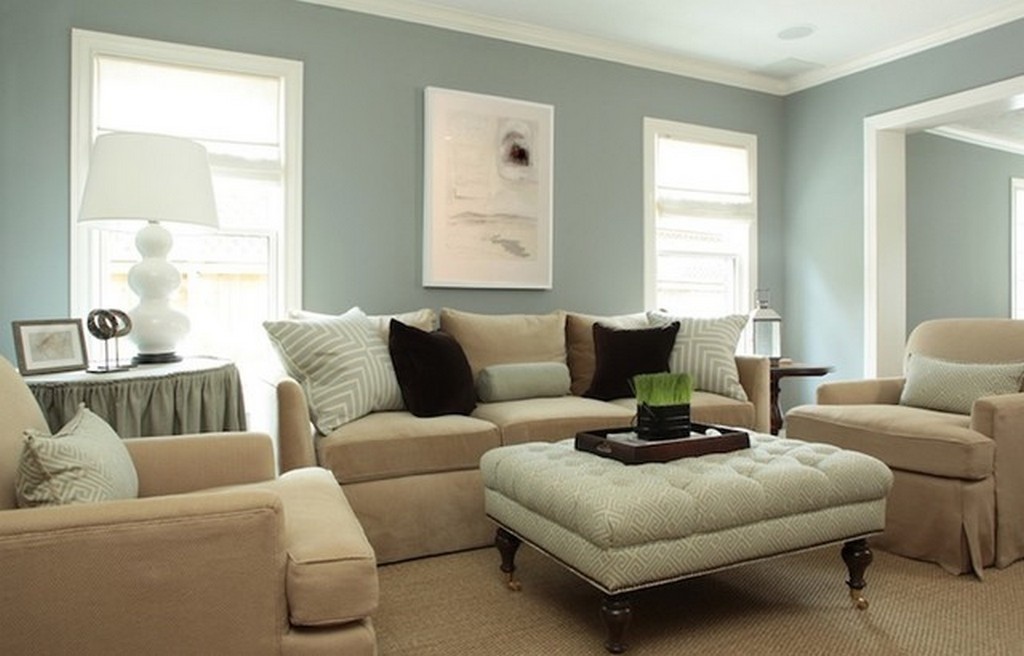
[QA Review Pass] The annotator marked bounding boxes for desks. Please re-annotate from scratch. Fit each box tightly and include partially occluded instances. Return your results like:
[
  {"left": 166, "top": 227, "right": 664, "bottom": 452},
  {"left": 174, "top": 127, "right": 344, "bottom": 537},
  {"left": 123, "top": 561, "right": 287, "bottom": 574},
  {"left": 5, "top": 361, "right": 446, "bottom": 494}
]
[
  {"left": 21, "top": 358, "right": 246, "bottom": 439},
  {"left": 770, "top": 365, "right": 827, "bottom": 435}
]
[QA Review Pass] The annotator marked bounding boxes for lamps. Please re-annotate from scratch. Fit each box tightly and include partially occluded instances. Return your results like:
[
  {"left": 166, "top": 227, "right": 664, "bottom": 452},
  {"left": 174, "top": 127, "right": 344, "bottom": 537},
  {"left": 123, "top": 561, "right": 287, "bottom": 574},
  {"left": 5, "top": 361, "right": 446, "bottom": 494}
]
[
  {"left": 76, "top": 132, "right": 223, "bottom": 367},
  {"left": 749, "top": 297, "right": 783, "bottom": 369}
]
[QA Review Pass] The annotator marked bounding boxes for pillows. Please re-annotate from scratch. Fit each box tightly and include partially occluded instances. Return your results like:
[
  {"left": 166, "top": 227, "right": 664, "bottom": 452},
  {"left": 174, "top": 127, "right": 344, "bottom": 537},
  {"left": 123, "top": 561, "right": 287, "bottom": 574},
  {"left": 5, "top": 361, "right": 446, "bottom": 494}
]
[
  {"left": 286, "top": 308, "right": 437, "bottom": 347},
  {"left": 262, "top": 307, "right": 407, "bottom": 436},
  {"left": 581, "top": 320, "right": 680, "bottom": 402},
  {"left": 568, "top": 313, "right": 650, "bottom": 395},
  {"left": 477, "top": 361, "right": 572, "bottom": 402},
  {"left": 648, "top": 309, "right": 751, "bottom": 402},
  {"left": 16, "top": 402, "right": 139, "bottom": 513},
  {"left": 898, "top": 353, "right": 1022, "bottom": 415},
  {"left": 438, "top": 308, "right": 568, "bottom": 392},
  {"left": 388, "top": 318, "right": 478, "bottom": 419}
]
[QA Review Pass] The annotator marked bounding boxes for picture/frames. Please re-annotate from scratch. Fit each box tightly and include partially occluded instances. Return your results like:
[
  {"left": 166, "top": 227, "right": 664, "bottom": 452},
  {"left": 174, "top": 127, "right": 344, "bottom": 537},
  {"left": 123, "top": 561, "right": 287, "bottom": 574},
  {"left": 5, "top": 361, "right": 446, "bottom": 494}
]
[
  {"left": 420, "top": 85, "right": 554, "bottom": 295},
  {"left": 12, "top": 319, "right": 89, "bottom": 377}
]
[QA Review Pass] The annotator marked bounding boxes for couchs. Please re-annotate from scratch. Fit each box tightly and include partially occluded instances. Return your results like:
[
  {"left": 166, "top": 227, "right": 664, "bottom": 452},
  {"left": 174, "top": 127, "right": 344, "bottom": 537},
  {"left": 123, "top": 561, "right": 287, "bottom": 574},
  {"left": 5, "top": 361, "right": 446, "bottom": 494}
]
[
  {"left": 784, "top": 319, "right": 1023, "bottom": 580},
  {"left": 278, "top": 350, "right": 770, "bottom": 566},
  {"left": 1, "top": 354, "right": 381, "bottom": 655}
]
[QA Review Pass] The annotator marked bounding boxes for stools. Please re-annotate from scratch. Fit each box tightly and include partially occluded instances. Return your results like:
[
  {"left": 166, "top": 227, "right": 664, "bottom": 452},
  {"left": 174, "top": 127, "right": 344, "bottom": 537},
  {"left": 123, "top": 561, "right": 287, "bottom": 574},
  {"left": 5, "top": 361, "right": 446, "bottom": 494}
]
[{"left": 480, "top": 423, "right": 894, "bottom": 653}]
